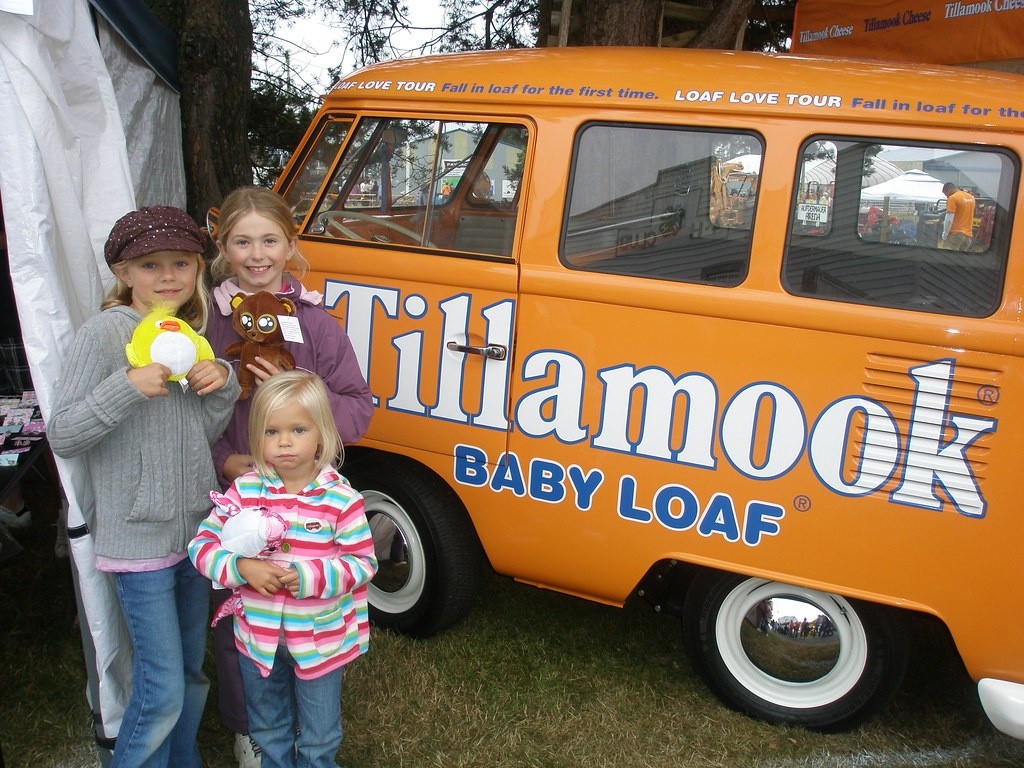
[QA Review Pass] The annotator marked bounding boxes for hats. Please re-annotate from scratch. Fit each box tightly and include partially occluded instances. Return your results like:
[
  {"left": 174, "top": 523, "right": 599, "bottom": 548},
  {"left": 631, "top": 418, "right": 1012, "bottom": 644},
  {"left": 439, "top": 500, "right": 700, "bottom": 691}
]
[{"left": 104, "top": 206, "right": 206, "bottom": 276}]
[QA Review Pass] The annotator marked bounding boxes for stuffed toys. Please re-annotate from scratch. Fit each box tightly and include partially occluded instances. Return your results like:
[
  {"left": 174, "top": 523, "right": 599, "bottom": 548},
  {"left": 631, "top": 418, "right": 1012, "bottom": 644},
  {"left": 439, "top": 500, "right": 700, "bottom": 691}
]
[
  {"left": 223, "top": 291, "right": 297, "bottom": 400},
  {"left": 206, "top": 487, "right": 291, "bottom": 560},
  {"left": 125, "top": 302, "right": 215, "bottom": 382}
]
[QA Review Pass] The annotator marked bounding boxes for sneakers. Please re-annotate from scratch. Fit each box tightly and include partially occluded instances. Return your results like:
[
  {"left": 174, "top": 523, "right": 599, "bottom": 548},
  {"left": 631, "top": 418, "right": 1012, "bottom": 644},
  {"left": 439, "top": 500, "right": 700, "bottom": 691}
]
[
  {"left": 0, "top": 499, "right": 32, "bottom": 528},
  {"left": 233, "top": 732, "right": 262, "bottom": 768},
  {"left": 51, "top": 508, "right": 68, "bottom": 559}
]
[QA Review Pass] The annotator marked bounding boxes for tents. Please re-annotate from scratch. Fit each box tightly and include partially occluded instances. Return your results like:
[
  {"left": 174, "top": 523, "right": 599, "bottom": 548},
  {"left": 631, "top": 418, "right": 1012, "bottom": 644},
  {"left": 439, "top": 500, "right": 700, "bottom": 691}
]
[{"left": 861, "top": 170, "right": 947, "bottom": 242}]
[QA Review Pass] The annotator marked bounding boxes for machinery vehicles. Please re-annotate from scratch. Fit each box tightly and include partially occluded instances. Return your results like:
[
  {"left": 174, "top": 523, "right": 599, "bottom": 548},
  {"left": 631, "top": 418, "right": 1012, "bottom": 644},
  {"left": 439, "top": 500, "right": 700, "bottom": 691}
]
[
  {"left": 799, "top": 181, "right": 996, "bottom": 254},
  {"left": 709, "top": 158, "right": 758, "bottom": 228}
]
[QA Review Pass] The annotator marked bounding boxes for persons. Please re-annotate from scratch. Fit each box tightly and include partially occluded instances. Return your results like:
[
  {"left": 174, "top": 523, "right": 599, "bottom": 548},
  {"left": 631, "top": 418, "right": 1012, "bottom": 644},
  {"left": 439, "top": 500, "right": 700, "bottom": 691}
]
[
  {"left": 332, "top": 179, "right": 379, "bottom": 206},
  {"left": 186, "top": 369, "right": 379, "bottom": 767},
  {"left": 441, "top": 182, "right": 451, "bottom": 204},
  {"left": 0, "top": 206, "right": 244, "bottom": 767},
  {"left": 798, "top": 189, "right": 831, "bottom": 206},
  {"left": 188, "top": 184, "right": 374, "bottom": 768},
  {"left": 942, "top": 182, "right": 976, "bottom": 251}
]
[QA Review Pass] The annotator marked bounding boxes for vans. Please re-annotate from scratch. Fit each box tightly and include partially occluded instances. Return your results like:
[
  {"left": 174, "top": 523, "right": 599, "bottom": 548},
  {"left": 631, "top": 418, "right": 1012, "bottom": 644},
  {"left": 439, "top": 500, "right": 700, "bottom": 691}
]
[{"left": 207, "top": 43, "right": 1023, "bottom": 743}]
